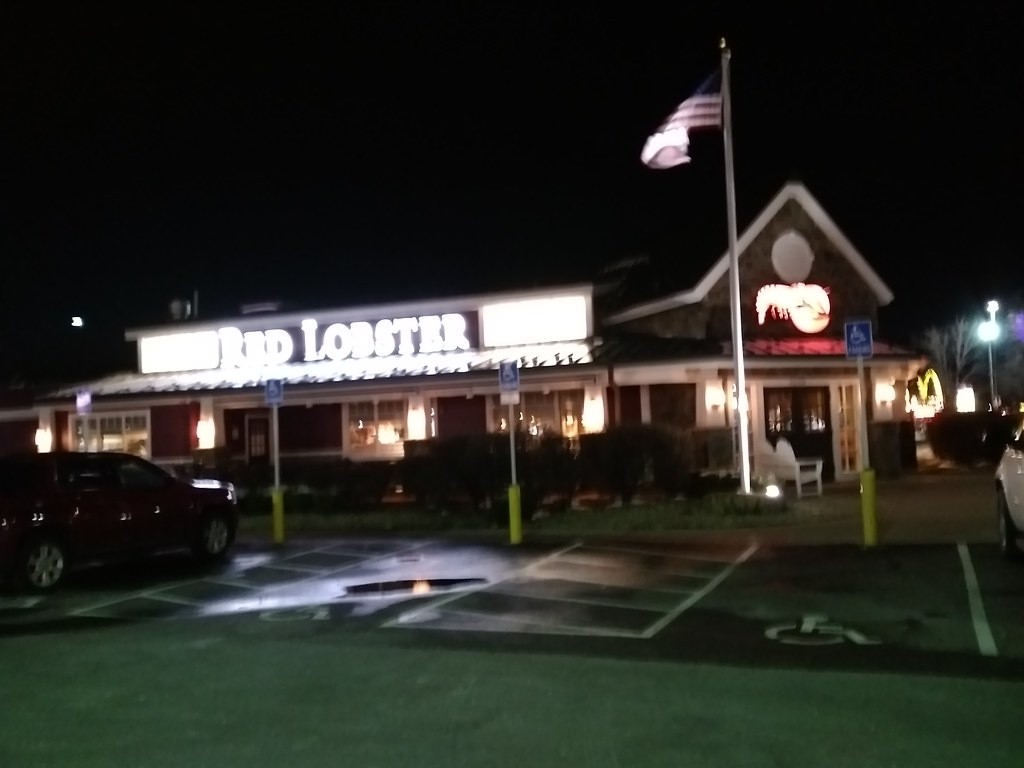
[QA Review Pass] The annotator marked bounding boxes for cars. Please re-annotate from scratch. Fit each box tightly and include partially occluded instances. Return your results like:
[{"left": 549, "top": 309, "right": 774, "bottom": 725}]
[
  {"left": 993, "top": 409, "right": 1024, "bottom": 563},
  {"left": 0, "top": 451, "right": 237, "bottom": 596}
]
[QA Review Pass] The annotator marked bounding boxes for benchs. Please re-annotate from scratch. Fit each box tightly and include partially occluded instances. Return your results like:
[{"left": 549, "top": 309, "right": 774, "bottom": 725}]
[{"left": 759, "top": 438, "right": 824, "bottom": 500}]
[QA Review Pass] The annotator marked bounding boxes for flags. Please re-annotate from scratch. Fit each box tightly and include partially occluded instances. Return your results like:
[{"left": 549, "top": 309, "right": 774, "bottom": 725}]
[{"left": 640, "top": 65, "right": 722, "bottom": 168}]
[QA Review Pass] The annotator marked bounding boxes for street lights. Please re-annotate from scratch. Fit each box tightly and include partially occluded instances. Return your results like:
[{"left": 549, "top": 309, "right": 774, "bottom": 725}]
[{"left": 976, "top": 319, "right": 1000, "bottom": 408}]
